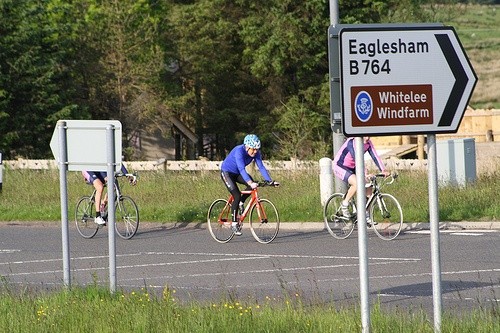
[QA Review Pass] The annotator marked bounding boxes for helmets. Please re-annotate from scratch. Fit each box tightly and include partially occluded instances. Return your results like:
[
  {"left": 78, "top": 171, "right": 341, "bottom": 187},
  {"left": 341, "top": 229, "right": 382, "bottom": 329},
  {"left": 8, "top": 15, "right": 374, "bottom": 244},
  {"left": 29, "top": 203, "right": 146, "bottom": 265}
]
[{"left": 243, "top": 134, "right": 261, "bottom": 149}]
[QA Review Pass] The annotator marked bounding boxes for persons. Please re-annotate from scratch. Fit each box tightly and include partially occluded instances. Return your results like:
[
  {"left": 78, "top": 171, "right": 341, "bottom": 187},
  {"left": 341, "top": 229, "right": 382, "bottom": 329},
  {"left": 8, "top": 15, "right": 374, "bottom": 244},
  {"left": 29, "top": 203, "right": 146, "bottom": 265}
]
[
  {"left": 332, "top": 136, "right": 389, "bottom": 225},
  {"left": 82, "top": 163, "right": 130, "bottom": 225},
  {"left": 221, "top": 134, "right": 277, "bottom": 236}
]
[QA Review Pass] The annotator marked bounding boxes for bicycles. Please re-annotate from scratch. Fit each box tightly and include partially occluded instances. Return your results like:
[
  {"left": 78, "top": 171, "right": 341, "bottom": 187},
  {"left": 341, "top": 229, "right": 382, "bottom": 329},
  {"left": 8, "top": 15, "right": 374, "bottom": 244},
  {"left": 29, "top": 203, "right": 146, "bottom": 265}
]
[
  {"left": 75, "top": 174, "right": 140, "bottom": 240},
  {"left": 207, "top": 180, "right": 280, "bottom": 244},
  {"left": 324, "top": 171, "right": 403, "bottom": 241}
]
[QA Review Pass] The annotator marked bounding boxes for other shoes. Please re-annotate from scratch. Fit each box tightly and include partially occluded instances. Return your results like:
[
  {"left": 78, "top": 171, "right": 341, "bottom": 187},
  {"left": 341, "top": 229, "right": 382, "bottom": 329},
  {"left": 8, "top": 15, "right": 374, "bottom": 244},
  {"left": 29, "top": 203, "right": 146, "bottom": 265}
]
[
  {"left": 231, "top": 224, "right": 242, "bottom": 235},
  {"left": 341, "top": 202, "right": 351, "bottom": 217},
  {"left": 95, "top": 216, "right": 105, "bottom": 225},
  {"left": 366, "top": 217, "right": 378, "bottom": 225}
]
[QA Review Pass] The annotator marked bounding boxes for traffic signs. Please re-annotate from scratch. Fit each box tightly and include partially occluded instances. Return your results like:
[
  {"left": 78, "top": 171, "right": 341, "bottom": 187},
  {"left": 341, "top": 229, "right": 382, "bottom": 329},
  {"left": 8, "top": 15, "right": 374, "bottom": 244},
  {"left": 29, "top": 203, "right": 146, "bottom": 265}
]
[{"left": 340, "top": 25, "right": 479, "bottom": 138}]
